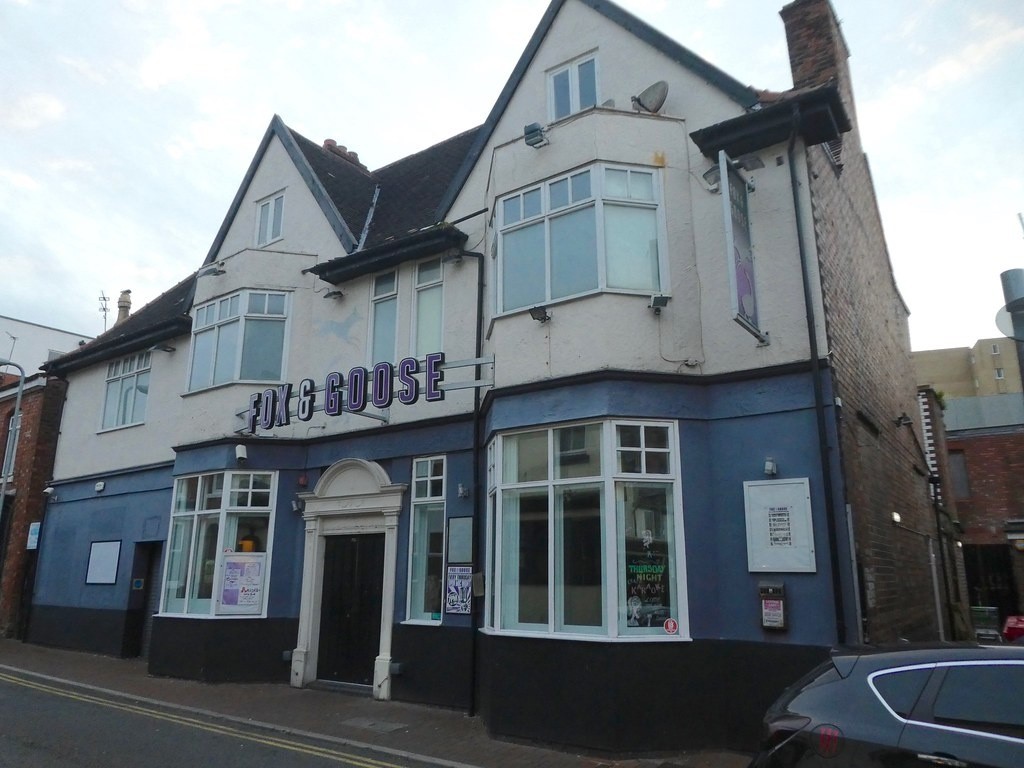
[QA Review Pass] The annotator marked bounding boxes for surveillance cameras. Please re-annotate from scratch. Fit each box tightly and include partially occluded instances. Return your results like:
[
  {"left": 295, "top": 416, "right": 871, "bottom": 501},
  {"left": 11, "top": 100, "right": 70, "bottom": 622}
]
[
  {"left": 43, "top": 488, "right": 54, "bottom": 496},
  {"left": 235, "top": 445, "right": 247, "bottom": 461}
]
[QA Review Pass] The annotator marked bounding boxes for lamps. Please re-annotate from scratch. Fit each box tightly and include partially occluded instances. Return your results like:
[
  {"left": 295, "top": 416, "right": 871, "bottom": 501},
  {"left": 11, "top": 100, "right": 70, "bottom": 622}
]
[
  {"left": 149, "top": 344, "right": 174, "bottom": 353},
  {"left": 323, "top": 291, "right": 344, "bottom": 299},
  {"left": 651, "top": 293, "right": 672, "bottom": 315},
  {"left": 196, "top": 268, "right": 225, "bottom": 276},
  {"left": 524, "top": 122, "right": 550, "bottom": 150},
  {"left": 529, "top": 305, "right": 551, "bottom": 323}
]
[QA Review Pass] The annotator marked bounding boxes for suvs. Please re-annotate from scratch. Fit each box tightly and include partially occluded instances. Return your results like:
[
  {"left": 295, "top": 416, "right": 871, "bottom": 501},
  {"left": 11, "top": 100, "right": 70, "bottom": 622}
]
[{"left": 744, "top": 631, "right": 1024, "bottom": 768}]
